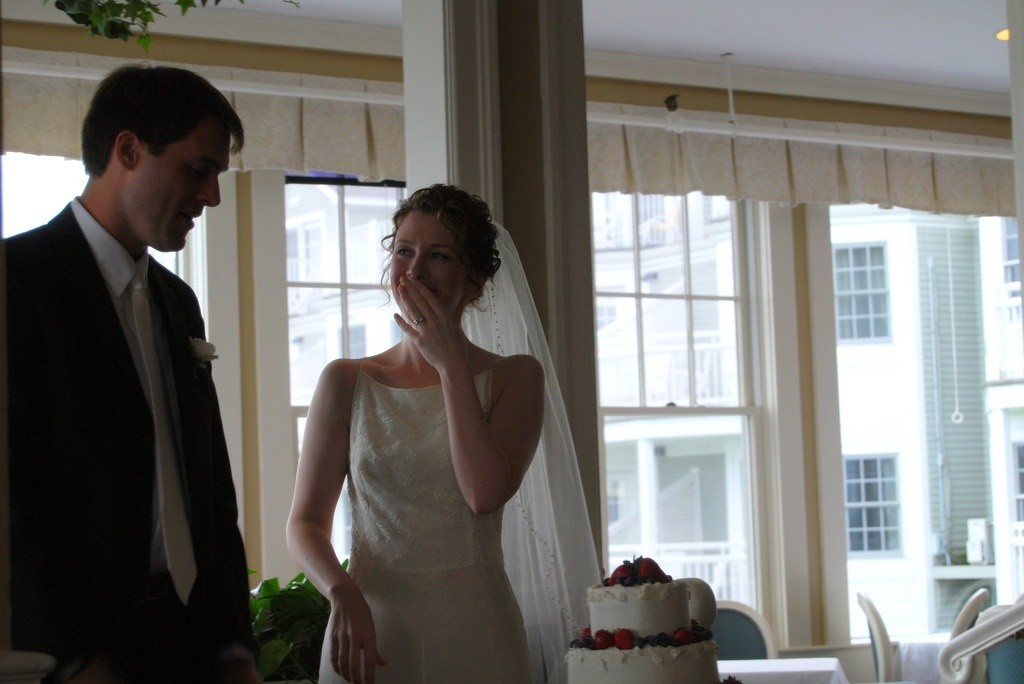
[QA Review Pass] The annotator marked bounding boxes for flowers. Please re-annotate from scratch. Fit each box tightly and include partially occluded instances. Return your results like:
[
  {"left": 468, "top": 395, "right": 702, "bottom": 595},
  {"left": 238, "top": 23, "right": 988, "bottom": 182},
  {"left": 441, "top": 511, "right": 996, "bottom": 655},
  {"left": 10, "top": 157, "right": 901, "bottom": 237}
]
[{"left": 188, "top": 337, "right": 219, "bottom": 371}]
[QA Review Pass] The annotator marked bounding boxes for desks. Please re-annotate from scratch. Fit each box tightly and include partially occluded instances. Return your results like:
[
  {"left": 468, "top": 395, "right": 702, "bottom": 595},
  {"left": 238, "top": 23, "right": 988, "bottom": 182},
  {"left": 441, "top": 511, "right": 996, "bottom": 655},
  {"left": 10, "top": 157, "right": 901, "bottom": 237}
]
[
  {"left": 717, "top": 657, "right": 849, "bottom": 684},
  {"left": 894, "top": 634, "right": 945, "bottom": 684}
]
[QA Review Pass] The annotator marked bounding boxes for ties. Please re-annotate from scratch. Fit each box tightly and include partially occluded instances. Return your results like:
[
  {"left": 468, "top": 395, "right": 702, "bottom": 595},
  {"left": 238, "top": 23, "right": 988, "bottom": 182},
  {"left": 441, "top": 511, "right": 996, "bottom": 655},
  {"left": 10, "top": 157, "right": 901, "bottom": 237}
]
[{"left": 128, "top": 266, "right": 198, "bottom": 605}]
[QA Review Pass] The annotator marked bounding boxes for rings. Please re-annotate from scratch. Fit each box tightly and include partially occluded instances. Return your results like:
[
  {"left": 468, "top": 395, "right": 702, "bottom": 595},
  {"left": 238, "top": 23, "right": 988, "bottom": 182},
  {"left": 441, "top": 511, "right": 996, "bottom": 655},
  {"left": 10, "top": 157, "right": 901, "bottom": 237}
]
[{"left": 414, "top": 317, "right": 423, "bottom": 325}]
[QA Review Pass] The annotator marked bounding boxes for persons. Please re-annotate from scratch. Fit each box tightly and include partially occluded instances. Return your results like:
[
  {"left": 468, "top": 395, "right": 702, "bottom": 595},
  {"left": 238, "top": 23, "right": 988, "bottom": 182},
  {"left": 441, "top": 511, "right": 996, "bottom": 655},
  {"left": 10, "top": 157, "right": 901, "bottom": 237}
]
[
  {"left": 0, "top": 65, "right": 266, "bottom": 684},
  {"left": 284, "top": 184, "right": 546, "bottom": 684}
]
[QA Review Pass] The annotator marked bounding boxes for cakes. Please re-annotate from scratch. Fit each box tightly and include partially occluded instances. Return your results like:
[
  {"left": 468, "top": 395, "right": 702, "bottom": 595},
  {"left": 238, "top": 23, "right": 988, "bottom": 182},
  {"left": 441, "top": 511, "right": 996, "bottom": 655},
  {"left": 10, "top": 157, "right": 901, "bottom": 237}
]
[{"left": 565, "top": 556, "right": 722, "bottom": 684}]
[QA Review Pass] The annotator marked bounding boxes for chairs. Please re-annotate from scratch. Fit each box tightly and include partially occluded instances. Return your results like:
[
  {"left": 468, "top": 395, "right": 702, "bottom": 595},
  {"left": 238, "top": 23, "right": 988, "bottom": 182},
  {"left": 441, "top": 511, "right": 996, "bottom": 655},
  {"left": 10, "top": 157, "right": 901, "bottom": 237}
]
[{"left": 709, "top": 587, "right": 1024, "bottom": 684}]
